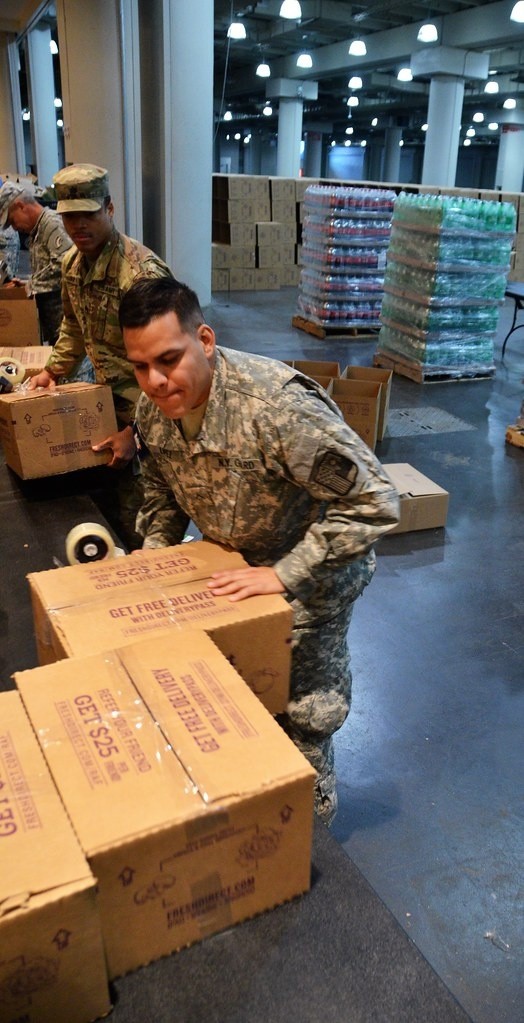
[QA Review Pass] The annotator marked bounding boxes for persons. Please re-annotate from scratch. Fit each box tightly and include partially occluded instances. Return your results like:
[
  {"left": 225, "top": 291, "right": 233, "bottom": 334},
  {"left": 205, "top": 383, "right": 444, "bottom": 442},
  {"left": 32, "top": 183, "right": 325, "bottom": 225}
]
[
  {"left": 118, "top": 276, "right": 400, "bottom": 831},
  {"left": 0, "top": 181, "right": 96, "bottom": 385},
  {"left": 25, "top": 164, "right": 174, "bottom": 553}
]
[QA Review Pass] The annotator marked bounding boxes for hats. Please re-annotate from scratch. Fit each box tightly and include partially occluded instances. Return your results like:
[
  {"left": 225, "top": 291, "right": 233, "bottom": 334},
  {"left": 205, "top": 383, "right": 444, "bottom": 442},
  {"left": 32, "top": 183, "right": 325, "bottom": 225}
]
[
  {"left": 0, "top": 181, "right": 25, "bottom": 227},
  {"left": 52, "top": 163, "right": 110, "bottom": 214}
]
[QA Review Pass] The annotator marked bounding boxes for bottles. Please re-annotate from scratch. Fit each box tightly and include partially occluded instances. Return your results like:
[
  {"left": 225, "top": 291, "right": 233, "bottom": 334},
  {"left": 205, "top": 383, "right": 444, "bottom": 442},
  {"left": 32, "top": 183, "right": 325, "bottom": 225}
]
[{"left": 298, "top": 184, "right": 516, "bottom": 366}]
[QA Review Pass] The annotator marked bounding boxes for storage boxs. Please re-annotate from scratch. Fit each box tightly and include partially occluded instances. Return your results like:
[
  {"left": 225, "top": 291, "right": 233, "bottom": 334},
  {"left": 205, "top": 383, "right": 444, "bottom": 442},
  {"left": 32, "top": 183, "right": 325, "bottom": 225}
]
[
  {"left": 212, "top": 173, "right": 524, "bottom": 293},
  {"left": 27, "top": 537, "right": 295, "bottom": 728},
  {"left": 1, "top": 382, "right": 118, "bottom": 479},
  {"left": 0, "top": 690, "right": 113, "bottom": 1023},
  {"left": 378, "top": 463, "right": 449, "bottom": 535},
  {"left": 281, "top": 360, "right": 392, "bottom": 455},
  {"left": 0, "top": 287, "right": 42, "bottom": 349},
  {"left": 18, "top": 625, "right": 318, "bottom": 983},
  {"left": 0, "top": 346, "right": 52, "bottom": 381}
]
